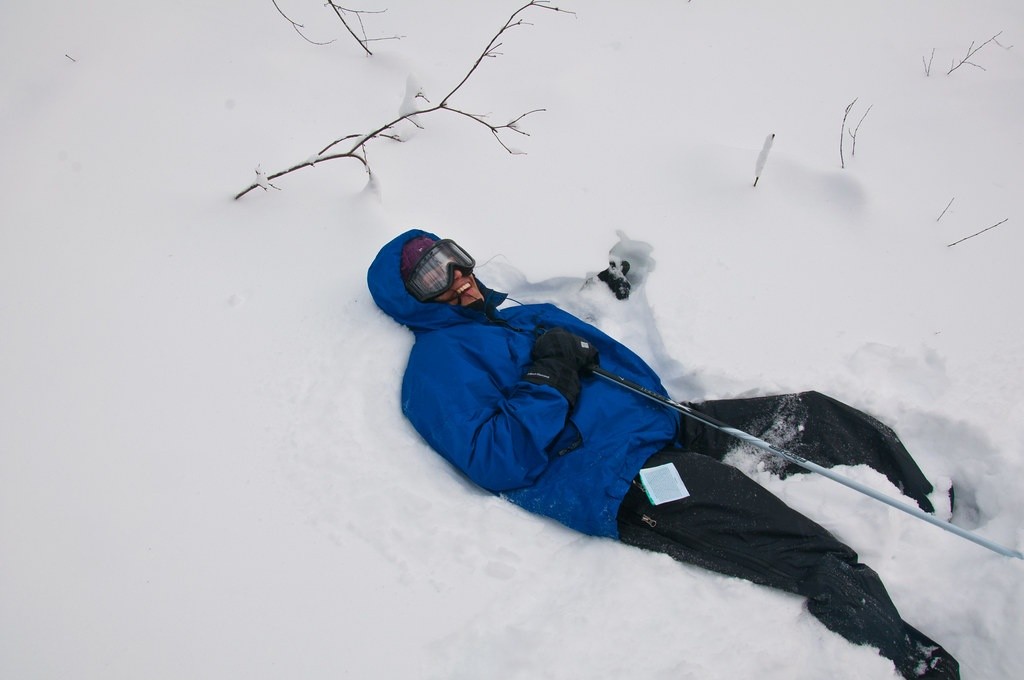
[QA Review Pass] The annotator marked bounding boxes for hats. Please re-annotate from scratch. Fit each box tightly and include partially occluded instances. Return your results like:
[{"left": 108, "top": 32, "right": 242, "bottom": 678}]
[{"left": 402, "top": 235, "right": 437, "bottom": 282}]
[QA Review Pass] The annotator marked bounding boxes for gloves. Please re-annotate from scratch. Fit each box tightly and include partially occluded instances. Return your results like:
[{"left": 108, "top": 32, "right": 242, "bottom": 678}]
[
  {"left": 522, "top": 326, "right": 601, "bottom": 415},
  {"left": 596, "top": 259, "right": 631, "bottom": 301}
]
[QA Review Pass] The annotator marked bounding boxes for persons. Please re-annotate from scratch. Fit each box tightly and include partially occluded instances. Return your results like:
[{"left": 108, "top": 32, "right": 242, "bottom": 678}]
[{"left": 366, "top": 229, "right": 963, "bottom": 680}]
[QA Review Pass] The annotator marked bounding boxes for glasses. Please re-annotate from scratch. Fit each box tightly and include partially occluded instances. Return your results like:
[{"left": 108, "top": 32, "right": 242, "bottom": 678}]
[{"left": 405, "top": 238, "right": 475, "bottom": 302}]
